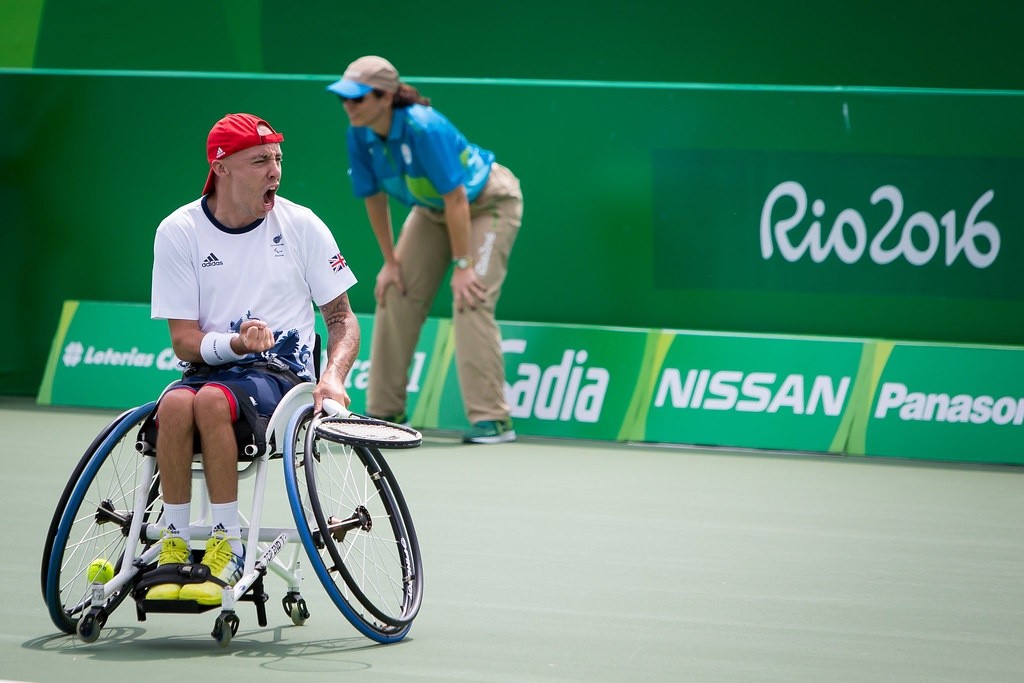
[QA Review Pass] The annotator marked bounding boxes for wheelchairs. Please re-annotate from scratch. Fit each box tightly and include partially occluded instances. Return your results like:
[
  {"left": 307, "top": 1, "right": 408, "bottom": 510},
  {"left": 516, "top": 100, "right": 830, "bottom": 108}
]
[{"left": 40, "top": 333, "right": 425, "bottom": 645}]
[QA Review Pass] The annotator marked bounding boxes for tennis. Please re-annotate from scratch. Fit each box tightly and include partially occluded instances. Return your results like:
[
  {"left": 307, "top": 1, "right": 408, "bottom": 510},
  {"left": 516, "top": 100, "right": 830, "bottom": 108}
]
[{"left": 87, "top": 558, "right": 115, "bottom": 585}]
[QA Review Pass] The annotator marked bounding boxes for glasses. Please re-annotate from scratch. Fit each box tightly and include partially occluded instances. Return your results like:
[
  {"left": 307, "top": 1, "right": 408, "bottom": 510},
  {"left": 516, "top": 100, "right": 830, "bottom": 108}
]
[{"left": 343, "top": 95, "right": 364, "bottom": 103}]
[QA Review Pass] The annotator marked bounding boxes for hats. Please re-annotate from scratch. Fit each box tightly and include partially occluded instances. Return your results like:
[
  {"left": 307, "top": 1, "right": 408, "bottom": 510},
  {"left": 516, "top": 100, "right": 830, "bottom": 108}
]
[
  {"left": 326, "top": 55, "right": 400, "bottom": 98},
  {"left": 202, "top": 113, "right": 284, "bottom": 195}
]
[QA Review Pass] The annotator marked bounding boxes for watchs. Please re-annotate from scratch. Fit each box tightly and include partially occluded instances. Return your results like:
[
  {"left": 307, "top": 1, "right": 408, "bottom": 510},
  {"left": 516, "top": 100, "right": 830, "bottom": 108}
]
[{"left": 451, "top": 255, "right": 473, "bottom": 270}]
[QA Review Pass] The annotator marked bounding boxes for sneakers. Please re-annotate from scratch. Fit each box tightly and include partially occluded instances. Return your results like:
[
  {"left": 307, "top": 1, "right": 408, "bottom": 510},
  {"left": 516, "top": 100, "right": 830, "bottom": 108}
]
[
  {"left": 145, "top": 529, "right": 194, "bottom": 599},
  {"left": 377, "top": 413, "right": 411, "bottom": 428},
  {"left": 462, "top": 421, "right": 517, "bottom": 444},
  {"left": 179, "top": 531, "right": 246, "bottom": 605}
]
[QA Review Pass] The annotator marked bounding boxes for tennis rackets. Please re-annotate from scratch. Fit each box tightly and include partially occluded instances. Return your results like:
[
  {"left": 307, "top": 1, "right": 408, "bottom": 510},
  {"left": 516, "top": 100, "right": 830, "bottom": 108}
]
[{"left": 313, "top": 398, "right": 423, "bottom": 450}]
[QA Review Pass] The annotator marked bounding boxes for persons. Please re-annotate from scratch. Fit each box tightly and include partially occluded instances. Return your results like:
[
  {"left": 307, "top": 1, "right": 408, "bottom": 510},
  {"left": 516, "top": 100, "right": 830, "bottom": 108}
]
[
  {"left": 139, "top": 113, "right": 360, "bottom": 605},
  {"left": 324, "top": 56, "right": 526, "bottom": 444}
]
